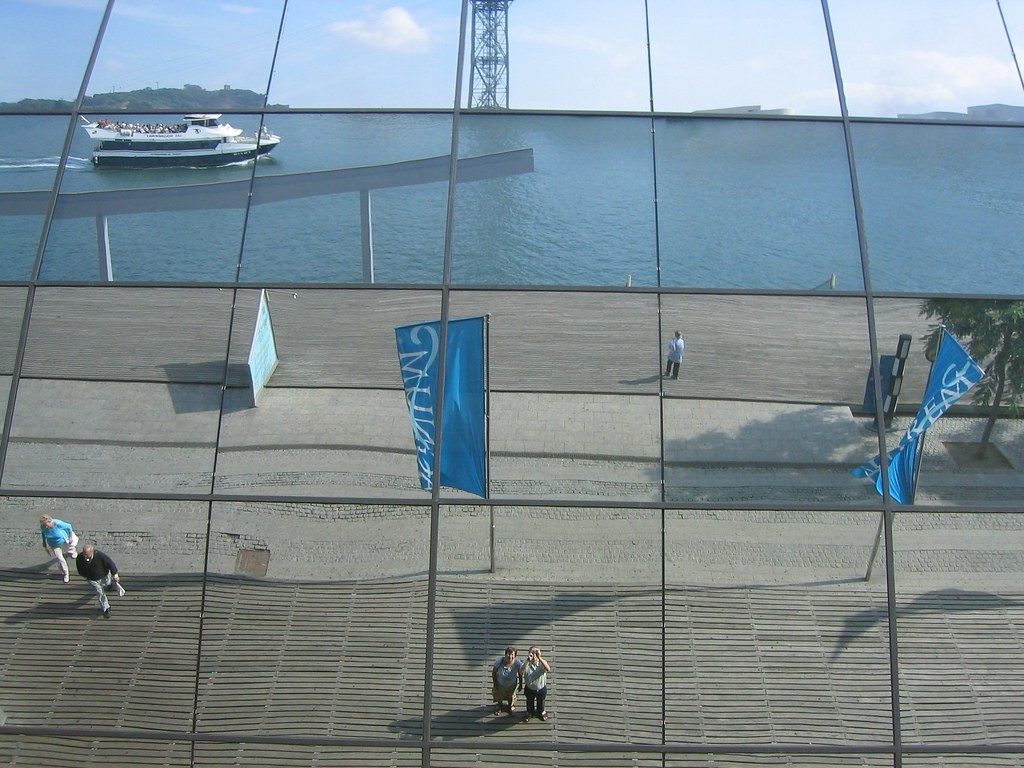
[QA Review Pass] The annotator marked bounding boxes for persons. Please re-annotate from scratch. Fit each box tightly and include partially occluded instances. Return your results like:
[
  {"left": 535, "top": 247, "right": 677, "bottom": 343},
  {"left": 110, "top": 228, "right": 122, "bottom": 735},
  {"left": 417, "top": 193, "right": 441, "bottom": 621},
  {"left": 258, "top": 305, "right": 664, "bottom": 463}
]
[
  {"left": 255, "top": 131, "right": 258, "bottom": 141},
  {"left": 98, "top": 119, "right": 187, "bottom": 133},
  {"left": 665, "top": 331, "right": 685, "bottom": 380},
  {"left": 492, "top": 647, "right": 523, "bottom": 716},
  {"left": 76, "top": 545, "right": 120, "bottom": 618},
  {"left": 520, "top": 647, "right": 550, "bottom": 721},
  {"left": 40, "top": 515, "right": 79, "bottom": 582},
  {"left": 262, "top": 124, "right": 267, "bottom": 136}
]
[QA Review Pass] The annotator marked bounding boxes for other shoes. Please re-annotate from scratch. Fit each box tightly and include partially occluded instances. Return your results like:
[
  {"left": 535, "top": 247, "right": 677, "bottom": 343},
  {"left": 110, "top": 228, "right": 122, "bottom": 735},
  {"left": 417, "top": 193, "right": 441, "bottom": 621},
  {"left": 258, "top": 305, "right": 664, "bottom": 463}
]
[
  {"left": 509, "top": 705, "right": 515, "bottom": 715},
  {"left": 107, "top": 585, "right": 113, "bottom": 592},
  {"left": 71, "top": 552, "right": 78, "bottom": 559},
  {"left": 64, "top": 573, "right": 69, "bottom": 583},
  {"left": 524, "top": 714, "right": 531, "bottom": 722},
  {"left": 104, "top": 606, "right": 111, "bottom": 618},
  {"left": 541, "top": 711, "right": 548, "bottom": 720},
  {"left": 495, "top": 704, "right": 502, "bottom": 715}
]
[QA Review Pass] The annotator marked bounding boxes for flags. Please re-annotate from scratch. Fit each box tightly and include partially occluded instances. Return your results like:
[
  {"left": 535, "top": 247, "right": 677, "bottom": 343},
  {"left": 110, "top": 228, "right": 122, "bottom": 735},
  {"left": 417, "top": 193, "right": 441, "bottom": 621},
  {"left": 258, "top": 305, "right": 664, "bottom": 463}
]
[
  {"left": 394, "top": 316, "right": 487, "bottom": 500},
  {"left": 858, "top": 331, "right": 985, "bottom": 507}
]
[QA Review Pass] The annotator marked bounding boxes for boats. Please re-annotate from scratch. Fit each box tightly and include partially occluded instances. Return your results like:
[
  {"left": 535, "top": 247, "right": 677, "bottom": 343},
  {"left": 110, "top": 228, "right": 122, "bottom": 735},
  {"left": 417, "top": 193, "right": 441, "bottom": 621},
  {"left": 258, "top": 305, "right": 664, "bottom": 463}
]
[{"left": 80, "top": 111, "right": 280, "bottom": 168}]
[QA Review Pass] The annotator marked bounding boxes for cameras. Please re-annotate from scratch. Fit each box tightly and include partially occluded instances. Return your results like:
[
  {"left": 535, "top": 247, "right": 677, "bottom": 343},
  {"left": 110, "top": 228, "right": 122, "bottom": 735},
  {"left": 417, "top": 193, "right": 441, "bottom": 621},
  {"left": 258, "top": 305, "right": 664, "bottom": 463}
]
[{"left": 531, "top": 650, "right": 537, "bottom": 653}]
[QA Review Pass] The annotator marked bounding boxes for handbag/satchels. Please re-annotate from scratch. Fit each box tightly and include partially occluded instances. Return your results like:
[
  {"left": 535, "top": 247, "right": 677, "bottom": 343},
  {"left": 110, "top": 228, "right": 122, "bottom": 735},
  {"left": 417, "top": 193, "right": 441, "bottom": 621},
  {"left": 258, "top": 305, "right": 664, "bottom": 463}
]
[
  {"left": 116, "top": 582, "right": 125, "bottom": 596},
  {"left": 57, "top": 521, "right": 79, "bottom": 548}
]
